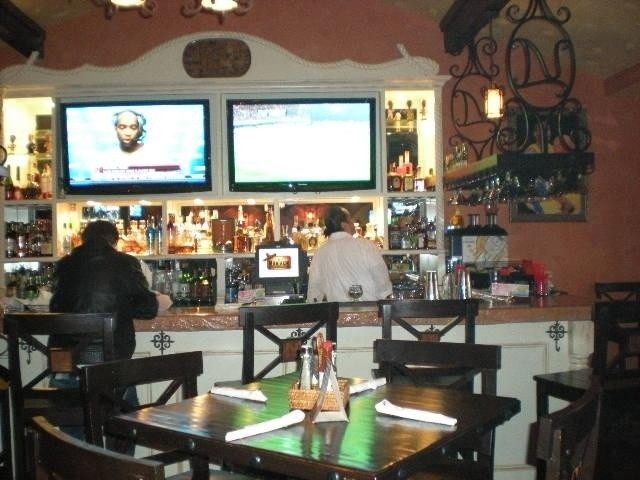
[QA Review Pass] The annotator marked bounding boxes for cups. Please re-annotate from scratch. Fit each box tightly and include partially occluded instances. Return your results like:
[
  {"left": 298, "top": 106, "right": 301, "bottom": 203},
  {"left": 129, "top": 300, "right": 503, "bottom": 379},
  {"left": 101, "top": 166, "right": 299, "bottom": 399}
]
[
  {"left": 535, "top": 279, "right": 548, "bottom": 298},
  {"left": 488, "top": 269, "right": 504, "bottom": 282}
]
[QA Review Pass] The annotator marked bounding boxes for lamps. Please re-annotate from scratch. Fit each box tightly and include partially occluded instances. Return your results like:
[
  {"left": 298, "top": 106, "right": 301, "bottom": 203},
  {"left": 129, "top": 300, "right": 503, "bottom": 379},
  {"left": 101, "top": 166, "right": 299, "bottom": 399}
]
[
  {"left": 482, "top": 11, "right": 506, "bottom": 120},
  {"left": 181, "top": 0, "right": 253, "bottom": 26},
  {"left": 95, "top": 0, "right": 158, "bottom": 20}
]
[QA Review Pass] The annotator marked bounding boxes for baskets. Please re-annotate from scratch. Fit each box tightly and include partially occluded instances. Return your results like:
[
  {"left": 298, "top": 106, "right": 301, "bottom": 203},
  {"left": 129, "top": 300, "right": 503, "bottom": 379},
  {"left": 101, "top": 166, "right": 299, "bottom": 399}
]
[{"left": 288, "top": 333, "right": 350, "bottom": 411}]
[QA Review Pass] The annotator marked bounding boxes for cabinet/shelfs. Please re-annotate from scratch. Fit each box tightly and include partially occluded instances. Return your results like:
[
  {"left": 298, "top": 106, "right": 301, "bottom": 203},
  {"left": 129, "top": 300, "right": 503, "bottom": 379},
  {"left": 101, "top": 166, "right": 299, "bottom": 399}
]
[{"left": 0, "top": 75, "right": 450, "bottom": 306}]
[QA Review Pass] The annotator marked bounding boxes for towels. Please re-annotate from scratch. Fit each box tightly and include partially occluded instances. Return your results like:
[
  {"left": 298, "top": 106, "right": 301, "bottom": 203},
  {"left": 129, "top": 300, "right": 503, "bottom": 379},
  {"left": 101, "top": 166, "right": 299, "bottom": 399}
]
[
  {"left": 223, "top": 409, "right": 305, "bottom": 445},
  {"left": 211, "top": 386, "right": 267, "bottom": 402},
  {"left": 375, "top": 399, "right": 457, "bottom": 427},
  {"left": 349, "top": 377, "right": 386, "bottom": 394}
]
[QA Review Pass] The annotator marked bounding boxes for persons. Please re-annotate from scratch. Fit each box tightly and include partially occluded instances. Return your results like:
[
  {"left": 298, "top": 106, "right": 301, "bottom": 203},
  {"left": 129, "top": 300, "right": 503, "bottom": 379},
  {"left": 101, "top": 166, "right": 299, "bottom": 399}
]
[
  {"left": 49, "top": 220, "right": 160, "bottom": 455},
  {"left": 305, "top": 207, "right": 393, "bottom": 303},
  {"left": 108, "top": 110, "right": 158, "bottom": 168}
]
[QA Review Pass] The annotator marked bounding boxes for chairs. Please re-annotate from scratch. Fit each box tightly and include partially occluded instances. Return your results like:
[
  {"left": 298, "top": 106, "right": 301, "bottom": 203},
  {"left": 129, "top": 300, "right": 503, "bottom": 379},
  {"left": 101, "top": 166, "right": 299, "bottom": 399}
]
[
  {"left": 3, "top": 311, "right": 117, "bottom": 480},
  {"left": 593, "top": 300, "right": 639, "bottom": 369},
  {"left": 375, "top": 338, "right": 501, "bottom": 480},
  {"left": 238, "top": 300, "right": 340, "bottom": 383},
  {"left": 595, "top": 282, "right": 640, "bottom": 302},
  {"left": 80, "top": 350, "right": 210, "bottom": 480},
  {"left": 32, "top": 415, "right": 164, "bottom": 480},
  {"left": 535, "top": 386, "right": 602, "bottom": 479},
  {"left": 372, "top": 298, "right": 479, "bottom": 379}
]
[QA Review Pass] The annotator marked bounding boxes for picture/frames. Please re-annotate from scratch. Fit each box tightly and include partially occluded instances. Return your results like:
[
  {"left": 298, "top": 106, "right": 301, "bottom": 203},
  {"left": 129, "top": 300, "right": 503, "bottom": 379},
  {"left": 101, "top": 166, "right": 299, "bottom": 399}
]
[{"left": 508, "top": 107, "right": 590, "bottom": 224}]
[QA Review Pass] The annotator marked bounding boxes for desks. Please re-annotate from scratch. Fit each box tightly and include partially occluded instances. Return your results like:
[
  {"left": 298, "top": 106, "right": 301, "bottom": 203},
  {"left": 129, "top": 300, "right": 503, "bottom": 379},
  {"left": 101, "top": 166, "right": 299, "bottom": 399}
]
[{"left": 534, "top": 369, "right": 639, "bottom": 480}]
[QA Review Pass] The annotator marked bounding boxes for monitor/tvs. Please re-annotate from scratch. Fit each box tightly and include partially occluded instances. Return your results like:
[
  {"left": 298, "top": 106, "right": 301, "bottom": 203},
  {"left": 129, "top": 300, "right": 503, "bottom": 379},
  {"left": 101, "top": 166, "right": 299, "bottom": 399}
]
[
  {"left": 225, "top": 97, "right": 378, "bottom": 192},
  {"left": 255, "top": 244, "right": 303, "bottom": 283},
  {"left": 59, "top": 99, "right": 213, "bottom": 196}
]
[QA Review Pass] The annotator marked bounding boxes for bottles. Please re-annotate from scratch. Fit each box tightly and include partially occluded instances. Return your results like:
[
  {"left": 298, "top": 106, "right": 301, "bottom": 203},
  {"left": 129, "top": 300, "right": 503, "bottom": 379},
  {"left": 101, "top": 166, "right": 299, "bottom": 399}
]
[
  {"left": 427, "top": 222, "right": 436, "bottom": 240},
  {"left": 28, "top": 134, "right": 38, "bottom": 154},
  {"left": 417, "top": 230, "right": 428, "bottom": 247},
  {"left": 280, "top": 216, "right": 384, "bottom": 250},
  {"left": 388, "top": 163, "right": 401, "bottom": 192},
  {"left": 402, "top": 150, "right": 413, "bottom": 174},
  {"left": 6, "top": 220, "right": 52, "bottom": 257},
  {"left": 318, "top": 341, "right": 336, "bottom": 390},
  {"left": 64, "top": 215, "right": 162, "bottom": 255},
  {"left": 7, "top": 134, "right": 17, "bottom": 154},
  {"left": 401, "top": 233, "right": 409, "bottom": 249},
  {"left": 239, "top": 269, "right": 250, "bottom": 292},
  {"left": 441, "top": 264, "right": 455, "bottom": 300},
  {"left": 426, "top": 269, "right": 438, "bottom": 299},
  {"left": 459, "top": 271, "right": 471, "bottom": 299},
  {"left": 167, "top": 206, "right": 273, "bottom": 254},
  {"left": 0, "top": 165, "right": 52, "bottom": 200},
  {"left": 460, "top": 142, "right": 468, "bottom": 166},
  {"left": 413, "top": 165, "right": 425, "bottom": 191},
  {"left": 425, "top": 168, "right": 435, "bottom": 191},
  {"left": 397, "top": 155, "right": 405, "bottom": 175},
  {"left": 452, "top": 258, "right": 463, "bottom": 286},
  {"left": 389, "top": 223, "right": 401, "bottom": 249},
  {"left": 5, "top": 264, "right": 52, "bottom": 300},
  {"left": 226, "top": 270, "right": 237, "bottom": 303},
  {"left": 454, "top": 146, "right": 461, "bottom": 167},
  {"left": 401, "top": 168, "right": 414, "bottom": 193},
  {"left": 299, "top": 339, "right": 314, "bottom": 390},
  {"left": 153, "top": 261, "right": 214, "bottom": 307}
]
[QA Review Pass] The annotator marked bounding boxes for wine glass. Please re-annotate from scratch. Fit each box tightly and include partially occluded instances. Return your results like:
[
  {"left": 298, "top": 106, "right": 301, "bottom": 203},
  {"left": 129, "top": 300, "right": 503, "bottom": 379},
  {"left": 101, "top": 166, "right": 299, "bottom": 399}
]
[{"left": 447, "top": 173, "right": 563, "bottom": 206}]
